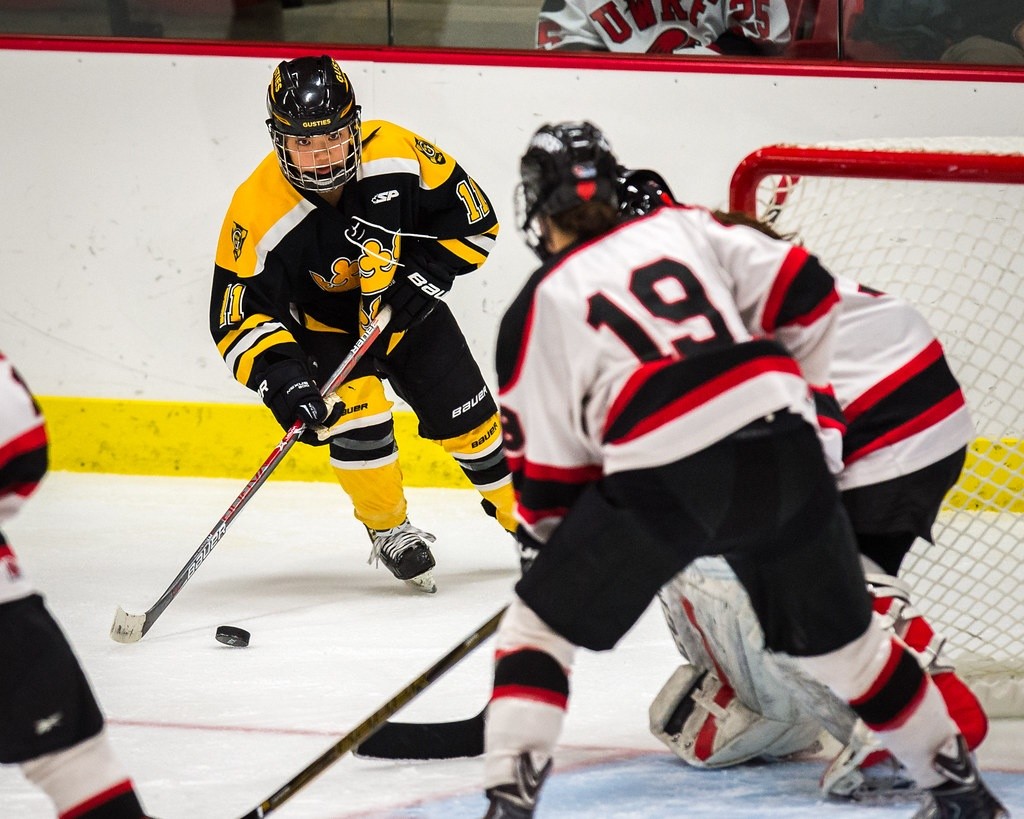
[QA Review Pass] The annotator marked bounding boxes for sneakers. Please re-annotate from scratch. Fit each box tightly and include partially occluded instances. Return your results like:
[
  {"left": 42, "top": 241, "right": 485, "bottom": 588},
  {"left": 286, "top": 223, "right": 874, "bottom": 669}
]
[{"left": 364, "top": 517, "right": 438, "bottom": 593}]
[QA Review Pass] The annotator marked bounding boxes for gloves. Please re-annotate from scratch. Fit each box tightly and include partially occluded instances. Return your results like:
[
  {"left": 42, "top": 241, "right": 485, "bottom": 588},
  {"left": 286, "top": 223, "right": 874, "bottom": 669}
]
[
  {"left": 378, "top": 257, "right": 455, "bottom": 333},
  {"left": 254, "top": 356, "right": 345, "bottom": 445}
]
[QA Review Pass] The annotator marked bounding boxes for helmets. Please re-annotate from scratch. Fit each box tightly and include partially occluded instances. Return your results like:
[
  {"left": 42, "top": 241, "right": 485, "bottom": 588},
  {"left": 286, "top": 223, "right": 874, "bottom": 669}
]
[
  {"left": 264, "top": 55, "right": 361, "bottom": 192},
  {"left": 614, "top": 161, "right": 673, "bottom": 214},
  {"left": 514, "top": 120, "right": 617, "bottom": 259}
]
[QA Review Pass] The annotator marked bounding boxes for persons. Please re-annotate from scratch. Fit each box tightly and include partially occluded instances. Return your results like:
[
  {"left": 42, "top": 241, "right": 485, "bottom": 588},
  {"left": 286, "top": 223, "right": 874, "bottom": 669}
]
[
  {"left": 863, "top": 0, "right": 1024, "bottom": 64},
  {"left": 208, "top": 55, "right": 538, "bottom": 593},
  {"left": 623, "top": 168, "right": 988, "bottom": 796},
  {"left": 0, "top": 353, "right": 152, "bottom": 819},
  {"left": 471, "top": 118, "right": 1011, "bottom": 819},
  {"left": 534, "top": 0, "right": 792, "bottom": 56}
]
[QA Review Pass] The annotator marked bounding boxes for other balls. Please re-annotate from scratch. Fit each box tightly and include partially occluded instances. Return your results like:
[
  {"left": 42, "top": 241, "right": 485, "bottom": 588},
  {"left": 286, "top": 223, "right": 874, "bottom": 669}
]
[{"left": 216, "top": 626, "right": 251, "bottom": 647}]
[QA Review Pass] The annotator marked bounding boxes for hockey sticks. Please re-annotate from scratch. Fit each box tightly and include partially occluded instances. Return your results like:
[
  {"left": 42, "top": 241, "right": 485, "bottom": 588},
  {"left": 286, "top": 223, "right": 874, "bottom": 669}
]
[
  {"left": 350, "top": 703, "right": 487, "bottom": 760},
  {"left": 243, "top": 598, "right": 514, "bottom": 819},
  {"left": 105, "top": 303, "right": 391, "bottom": 645}
]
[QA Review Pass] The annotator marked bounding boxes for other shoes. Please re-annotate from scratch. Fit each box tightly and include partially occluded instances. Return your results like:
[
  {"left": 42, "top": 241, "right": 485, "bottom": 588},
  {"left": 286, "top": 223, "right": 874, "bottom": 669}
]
[
  {"left": 819, "top": 741, "right": 919, "bottom": 801},
  {"left": 481, "top": 751, "right": 553, "bottom": 819},
  {"left": 930, "top": 735, "right": 1010, "bottom": 819}
]
